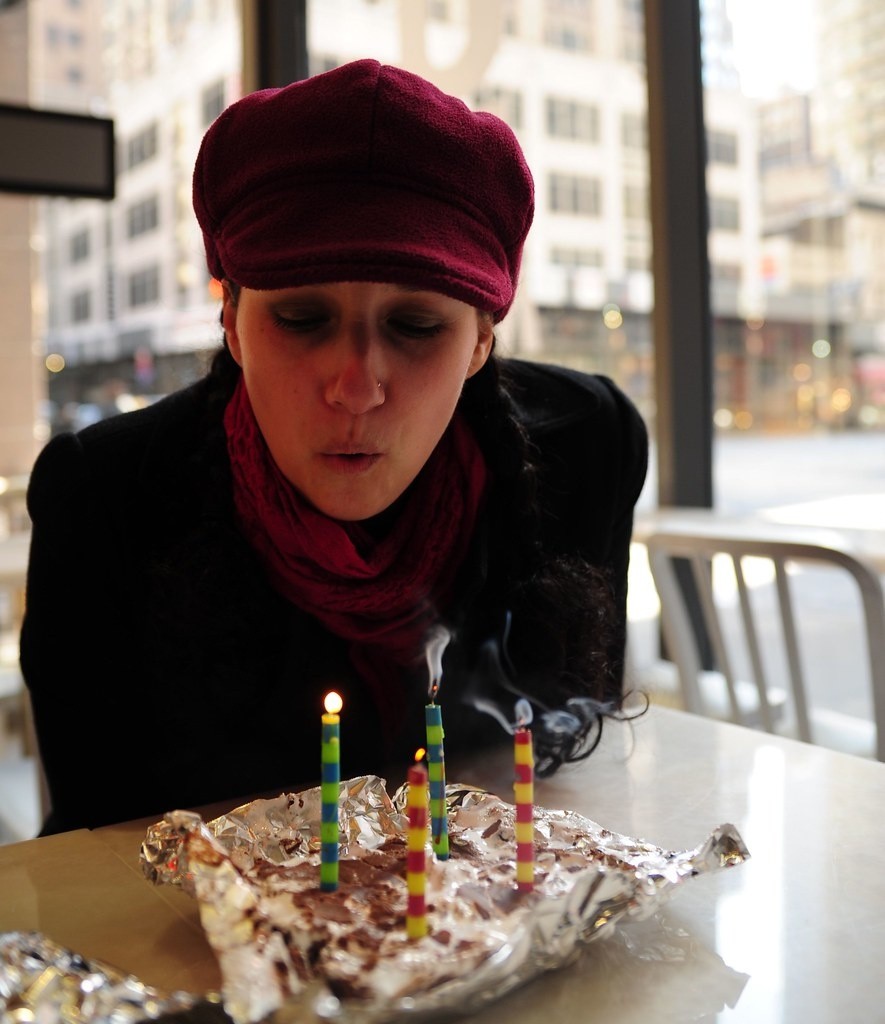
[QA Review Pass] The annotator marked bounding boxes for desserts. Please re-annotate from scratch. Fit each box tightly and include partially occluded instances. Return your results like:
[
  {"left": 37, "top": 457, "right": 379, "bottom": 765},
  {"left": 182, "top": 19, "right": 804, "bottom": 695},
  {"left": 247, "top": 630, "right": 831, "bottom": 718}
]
[{"left": 249, "top": 823, "right": 581, "bottom": 1004}]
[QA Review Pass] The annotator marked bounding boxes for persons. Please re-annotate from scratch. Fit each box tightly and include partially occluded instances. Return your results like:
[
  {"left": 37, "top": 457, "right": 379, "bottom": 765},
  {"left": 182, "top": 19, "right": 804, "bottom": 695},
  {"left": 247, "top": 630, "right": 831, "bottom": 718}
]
[{"left": 20, "top": 58, "right": 647, "bottom": 837}]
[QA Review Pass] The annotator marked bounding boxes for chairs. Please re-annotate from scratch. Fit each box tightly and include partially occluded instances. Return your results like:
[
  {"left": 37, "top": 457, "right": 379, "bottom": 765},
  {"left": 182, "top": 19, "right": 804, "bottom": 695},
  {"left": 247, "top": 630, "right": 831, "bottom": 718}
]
[{"left": 623, "top": 508, "right": 885, "bottom": 757}]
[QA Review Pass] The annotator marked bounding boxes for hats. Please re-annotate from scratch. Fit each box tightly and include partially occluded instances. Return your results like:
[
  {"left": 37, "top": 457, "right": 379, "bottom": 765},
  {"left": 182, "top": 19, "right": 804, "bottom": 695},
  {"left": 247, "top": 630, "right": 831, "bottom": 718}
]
[{"left": 193, "top": 57, "right": 534, "bottom": 321}]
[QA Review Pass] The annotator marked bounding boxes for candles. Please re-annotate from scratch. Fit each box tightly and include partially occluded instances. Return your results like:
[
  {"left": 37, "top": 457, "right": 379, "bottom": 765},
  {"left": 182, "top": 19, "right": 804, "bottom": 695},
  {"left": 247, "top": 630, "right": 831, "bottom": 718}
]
[
  {"left": 407, "top": 747, "right": 427, "bottom": 945},
  {"left": 425, "top": 675, "right": 449, "bottom": 863},
  {"left": 513, "top": 714, "right": 535, "bottom": 895},
  {"left": 321, "top": 691, "right": 343, "bottom": 894}
]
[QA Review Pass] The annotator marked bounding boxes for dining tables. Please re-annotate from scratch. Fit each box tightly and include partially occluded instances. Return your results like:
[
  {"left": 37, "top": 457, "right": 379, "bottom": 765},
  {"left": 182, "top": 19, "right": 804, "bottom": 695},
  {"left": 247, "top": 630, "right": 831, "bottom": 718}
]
[{"left": 1, "top": 704, "right": 885, "bottom": 1023}]
